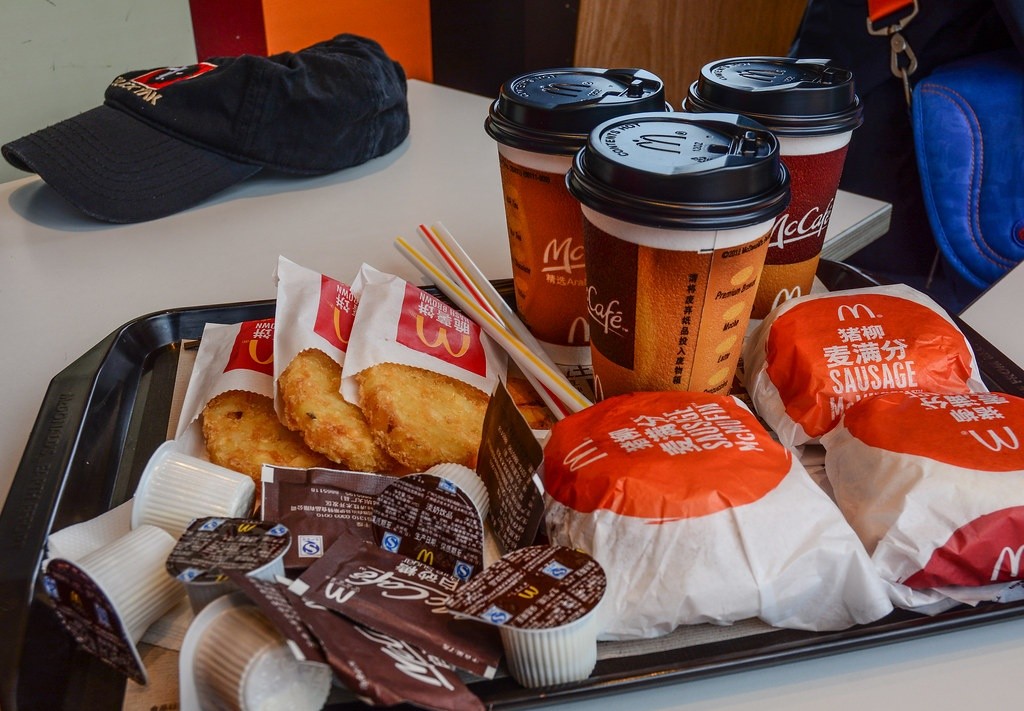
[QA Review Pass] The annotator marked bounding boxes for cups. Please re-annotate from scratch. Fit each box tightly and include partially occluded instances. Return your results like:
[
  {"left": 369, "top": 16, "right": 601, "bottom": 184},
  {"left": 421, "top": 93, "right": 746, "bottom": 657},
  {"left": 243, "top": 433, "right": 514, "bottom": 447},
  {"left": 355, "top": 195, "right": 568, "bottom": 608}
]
[
  {"left": 130, "top": 441, "right": 256, "bottom": 539},
  {"left": 178, "top": 597, "right": 331, "bottom": 710},
  {"left": 45, "top": 524, "right": 186, "bottom": 685},
  {"left": 484, "top": 68, "right": 675, "bottom": 366},
  {"left": 447, "top": 543, "right": 606, "bottom": 692},
  {"left": 563, "top": 112, "right": 791, "bottom": 398},
  {"left": 372, "top": 463, "right": 490, "bottom": 577},
  {"left": 683, "top": 56, "right": 866, "bottom": 337},
  {"left": 165, "top": 515, "right": 292, "bottom": 612}
]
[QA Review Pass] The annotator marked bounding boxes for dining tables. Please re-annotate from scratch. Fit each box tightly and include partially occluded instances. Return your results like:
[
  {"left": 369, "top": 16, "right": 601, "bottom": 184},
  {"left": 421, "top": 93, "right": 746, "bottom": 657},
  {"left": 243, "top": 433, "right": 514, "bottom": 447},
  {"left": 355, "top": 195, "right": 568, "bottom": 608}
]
[{"left": 0, "top": 78, "right": 1024, "bottom": 710}]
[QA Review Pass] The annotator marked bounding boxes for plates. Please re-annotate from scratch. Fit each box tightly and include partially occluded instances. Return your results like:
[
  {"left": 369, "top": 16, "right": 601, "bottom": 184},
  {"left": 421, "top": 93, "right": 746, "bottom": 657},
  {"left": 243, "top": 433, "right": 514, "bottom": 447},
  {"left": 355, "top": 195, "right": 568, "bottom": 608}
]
[{"left": 0, "top": 256, "right": 1024, "bottom": 710}]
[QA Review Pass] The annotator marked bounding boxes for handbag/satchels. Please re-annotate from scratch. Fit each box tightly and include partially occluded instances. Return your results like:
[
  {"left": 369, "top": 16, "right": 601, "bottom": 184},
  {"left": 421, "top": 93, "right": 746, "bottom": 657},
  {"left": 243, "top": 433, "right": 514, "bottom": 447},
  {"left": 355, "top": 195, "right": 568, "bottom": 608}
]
[{"left": 866, "top": 0, "right": 1024, "bottom": 289}]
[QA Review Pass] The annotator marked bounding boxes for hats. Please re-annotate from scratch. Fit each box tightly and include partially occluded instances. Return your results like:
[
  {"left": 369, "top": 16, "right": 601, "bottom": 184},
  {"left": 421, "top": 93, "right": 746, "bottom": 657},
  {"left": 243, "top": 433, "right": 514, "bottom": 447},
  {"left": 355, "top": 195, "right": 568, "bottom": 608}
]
[{"left": 1, "top": 34, "right": 410, "bottom": 222}]
